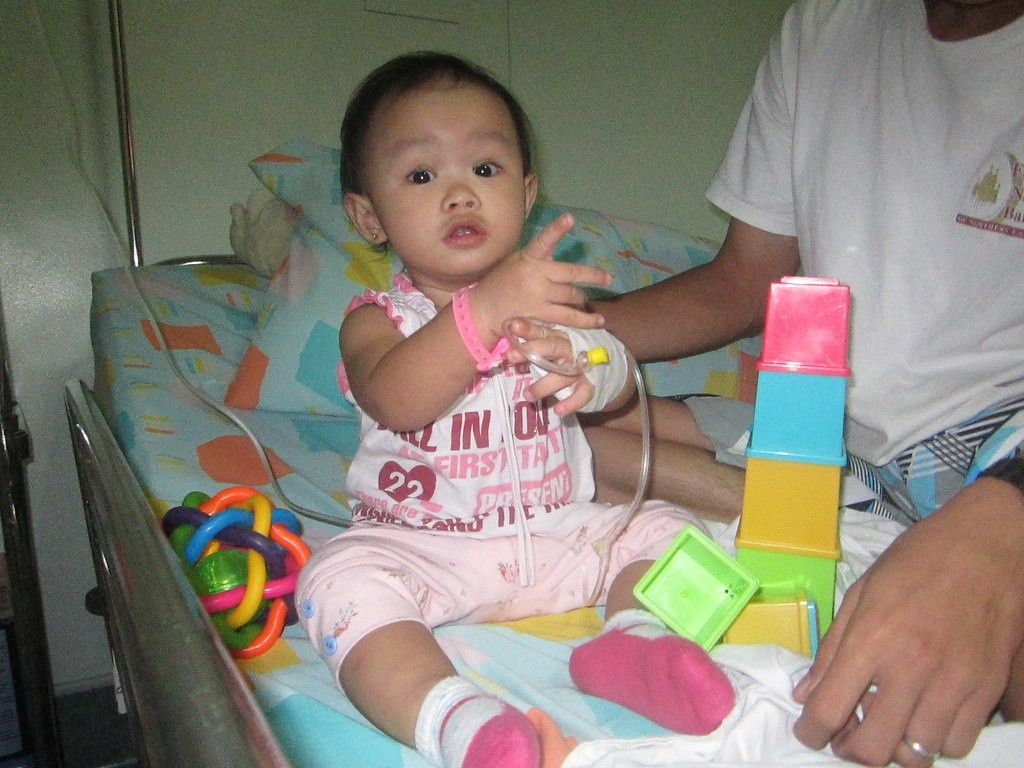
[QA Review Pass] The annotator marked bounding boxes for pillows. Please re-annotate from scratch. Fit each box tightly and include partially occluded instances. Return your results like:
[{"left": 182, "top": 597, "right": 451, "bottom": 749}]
[{"left": 224, "top": 140, "right": 768, "bottom": 418}]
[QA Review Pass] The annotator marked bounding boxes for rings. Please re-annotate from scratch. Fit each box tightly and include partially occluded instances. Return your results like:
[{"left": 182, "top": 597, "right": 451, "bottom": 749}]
[{"left": 903, "top": 735, "right": 941, "bottom": 761}]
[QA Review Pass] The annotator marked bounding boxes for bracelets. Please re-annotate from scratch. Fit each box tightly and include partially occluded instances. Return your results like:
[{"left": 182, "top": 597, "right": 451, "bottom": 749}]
[{"left": 451, "top": 286, "right": 510, "bottom": 376}]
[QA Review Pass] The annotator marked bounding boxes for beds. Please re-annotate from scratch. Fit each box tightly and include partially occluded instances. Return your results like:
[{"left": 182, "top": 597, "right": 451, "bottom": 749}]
[{"left": 64, "top": 255, "right": 1024, "bottom": 768}]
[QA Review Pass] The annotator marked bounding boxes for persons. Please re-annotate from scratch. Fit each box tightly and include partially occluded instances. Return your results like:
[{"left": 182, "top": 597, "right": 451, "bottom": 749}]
[
  {"left": 291, "top": 51, "right": 738, "bottom": 767},
  {"left": 571, "top": 2, "right": 1024, "bottom": 768}
]
